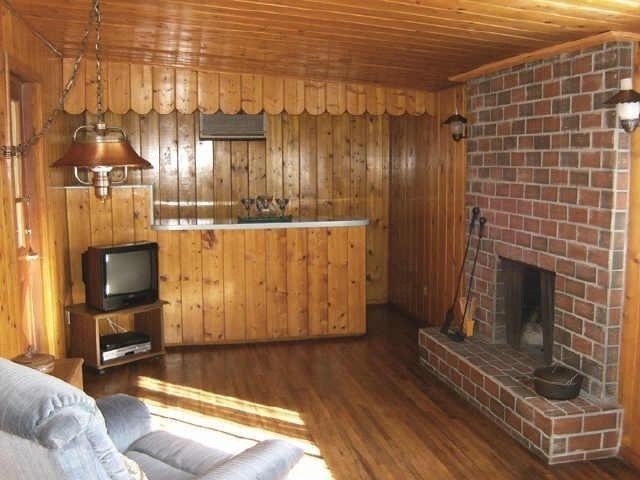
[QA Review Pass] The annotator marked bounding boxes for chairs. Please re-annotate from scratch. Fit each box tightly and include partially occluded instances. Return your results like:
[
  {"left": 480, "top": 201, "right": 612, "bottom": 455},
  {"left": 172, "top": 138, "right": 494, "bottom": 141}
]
[{"left": 0, "top": 357, "right": 305, "bottom": 480}]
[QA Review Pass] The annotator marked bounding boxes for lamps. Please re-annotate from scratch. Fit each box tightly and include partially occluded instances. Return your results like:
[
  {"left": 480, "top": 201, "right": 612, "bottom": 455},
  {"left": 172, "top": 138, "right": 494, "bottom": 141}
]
[
  {"left": 441, "top": 112, "right": 471, "bottom": 142},
  {"left": 0, "top": 0, "right": 154, "bottom": 203},
  {"left": 9, "top": 198, "right": 57, "bottom": 373},
  {"left": 601, "top": 79, "right": 640, "bottom": 135}
]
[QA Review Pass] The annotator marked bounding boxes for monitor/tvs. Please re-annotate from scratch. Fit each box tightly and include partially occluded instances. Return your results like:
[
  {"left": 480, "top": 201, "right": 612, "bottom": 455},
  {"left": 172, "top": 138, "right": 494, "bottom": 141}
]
[{"left": 81, "top": 240, "right": 160, "bottom": 313}]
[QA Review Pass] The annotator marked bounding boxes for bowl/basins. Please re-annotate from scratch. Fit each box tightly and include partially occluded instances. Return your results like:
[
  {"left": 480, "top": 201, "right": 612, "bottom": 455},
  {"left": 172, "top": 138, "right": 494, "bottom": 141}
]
[{"left": 532, "top": 366, "right": 583, "bottom": 400}]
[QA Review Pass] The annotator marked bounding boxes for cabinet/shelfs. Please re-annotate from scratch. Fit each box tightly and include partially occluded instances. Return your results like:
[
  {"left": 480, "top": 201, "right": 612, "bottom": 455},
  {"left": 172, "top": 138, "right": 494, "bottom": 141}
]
[
  {"left": 62, "top": 297, "right": 169, "bottom": 376},
  {"left": 52, "top": 357, "right": 86, "bottom": 392}
]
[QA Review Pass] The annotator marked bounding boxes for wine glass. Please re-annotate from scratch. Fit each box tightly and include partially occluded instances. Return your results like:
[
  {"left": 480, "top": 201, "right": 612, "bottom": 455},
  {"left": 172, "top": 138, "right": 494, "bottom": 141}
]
[
  {"left": 275, "top": 198, "right": 288, "bottom": 216},
  {"left": 241, "top": 199, "right": 254, "bottom": 217}
]
